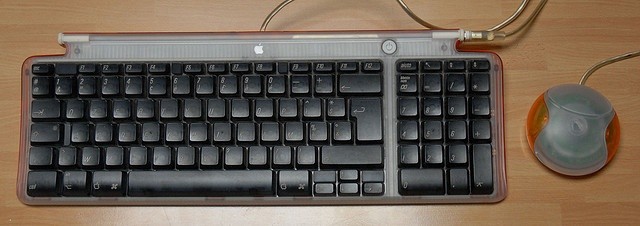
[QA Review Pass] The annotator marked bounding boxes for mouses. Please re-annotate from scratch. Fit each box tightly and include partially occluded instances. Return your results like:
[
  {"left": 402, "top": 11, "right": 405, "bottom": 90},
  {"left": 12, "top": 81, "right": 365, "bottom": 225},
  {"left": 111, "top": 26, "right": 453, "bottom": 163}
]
[{"left": 526, "top": 82, "right": 621, "bottom": 178}]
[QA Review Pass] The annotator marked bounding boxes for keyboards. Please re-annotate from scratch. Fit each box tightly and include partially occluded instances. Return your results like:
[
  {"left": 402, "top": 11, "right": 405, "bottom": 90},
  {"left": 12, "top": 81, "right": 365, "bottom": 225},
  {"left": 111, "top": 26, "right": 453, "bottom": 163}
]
[{"left": 15, "top": 29, "right": 509, "bottom": 207}]
